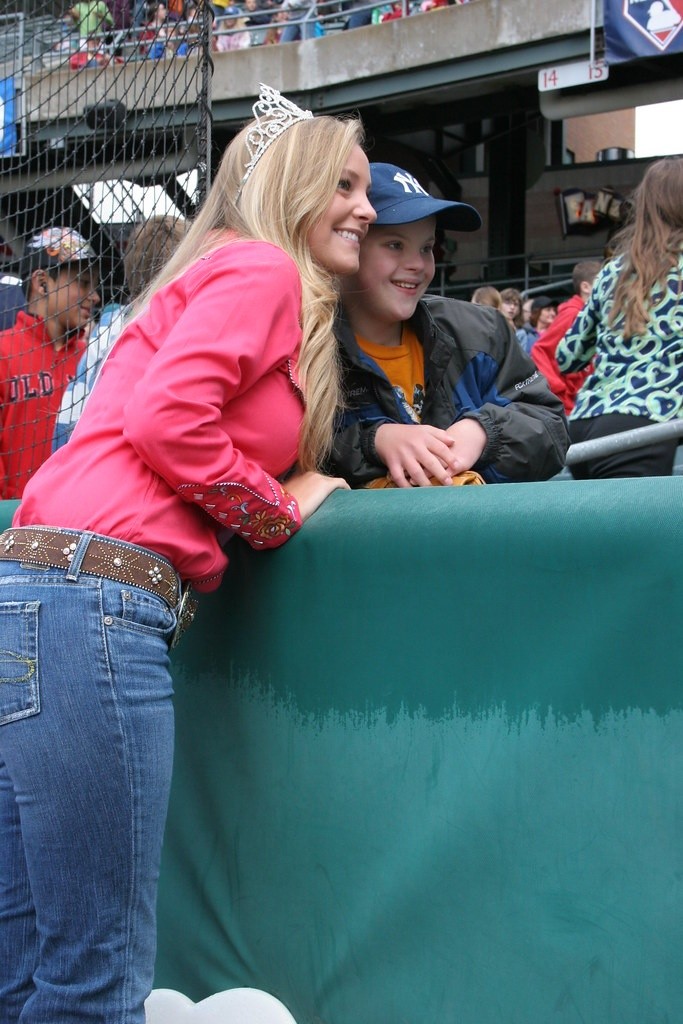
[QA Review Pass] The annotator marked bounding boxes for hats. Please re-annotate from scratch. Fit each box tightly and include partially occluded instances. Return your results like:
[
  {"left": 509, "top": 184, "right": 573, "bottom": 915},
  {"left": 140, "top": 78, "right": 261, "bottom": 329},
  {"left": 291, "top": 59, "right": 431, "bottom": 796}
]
[
  {"left": 370, "top": 162, "right": 483, "bottom": 237},
  {"left": 530, "top": 295, "right": 558, "bottom": 312},
  {"left": 19, "top": 225, "right": 122, "bottom": 281},
  {"left": 223, "top": 6, "right": 239, "bottom": 16}
]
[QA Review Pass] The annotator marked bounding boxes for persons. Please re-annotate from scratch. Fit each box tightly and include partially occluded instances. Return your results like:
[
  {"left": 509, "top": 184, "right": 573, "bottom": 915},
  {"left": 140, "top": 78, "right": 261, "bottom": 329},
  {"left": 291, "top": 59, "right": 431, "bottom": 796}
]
[
  {"left": 70, "top": 0, "right": 463, "bottom": 70},
  {"left": 285, "top": 162, "right": 572, "bottom": 492},
  {"left": 472, "top": 256, "right": 606, "bottom": 420},
  {"left": 0, "top": 112, "right": 380, "bottom": 1024},
  {"left": 0, "top": 214, "right": 194, "bottom": 504},
  {"left": 551, "top": 155, "right": 682, "bottom": 481}
]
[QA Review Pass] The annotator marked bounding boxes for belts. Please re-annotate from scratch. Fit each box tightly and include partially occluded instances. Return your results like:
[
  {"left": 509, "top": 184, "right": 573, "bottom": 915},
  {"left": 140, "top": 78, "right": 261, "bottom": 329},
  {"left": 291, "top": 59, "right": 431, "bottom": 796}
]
[{"left": 1, "top": 523, "right": 203, "bottom": 650}]
